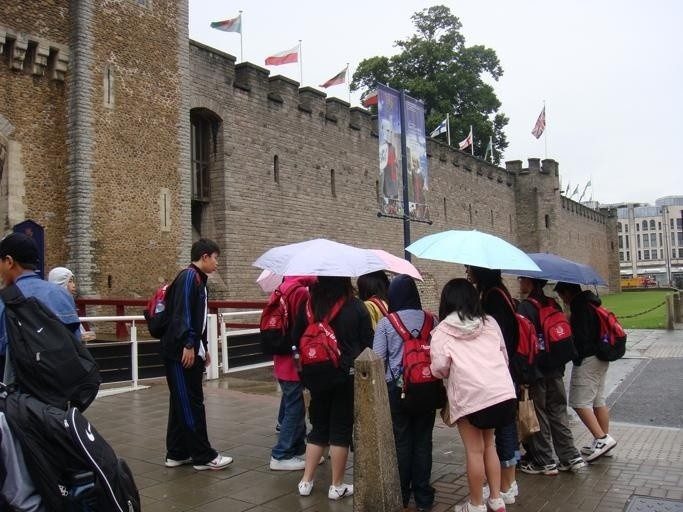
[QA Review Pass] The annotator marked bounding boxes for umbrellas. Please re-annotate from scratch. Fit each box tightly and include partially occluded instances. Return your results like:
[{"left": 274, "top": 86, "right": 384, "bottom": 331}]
[
  {"left": 404, "top": 229, "right": 541, "bottom": 289},
  {"left": 256, "top": 268, "right": 283, "bottom": 294},
  {"left": 251, "top": 237, "right": 387, "bottom": 278},
  {"left": 501, "top": 253, "right": 606, "bottom": 298},
  {"left": 367, "top": 247, "right": 424, "bottom": 283}
]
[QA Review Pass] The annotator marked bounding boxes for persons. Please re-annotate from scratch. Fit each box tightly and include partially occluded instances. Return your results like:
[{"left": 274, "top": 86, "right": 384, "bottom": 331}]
[
  {"left": 297, "top": 276, "right": 372, "bottom": 501},
  {"left": 463, "top": 264, "right": 520, "bottom": 504},
  {"left": 275, "top": 350, "right": 306, "bottom": 434},
  {"left": 371, "top": 274, "right": 440, "bottom": 511},
  {"left": 356, "top": 269, "right": 390, "bottom": 334},
  {"left": 551, "top": 281, "right": 617, "bottom": 463},
  {"left": 517, "top": 290, "right": 530, "bottom": 304},
  {"left": 47, "top": 266, "right": 94, "bottom": 346},
  {"left": 519, "top": 277, "right": 585, "bottom": 477},
  {"left": 267, "top": 275, "right": 324, "bottom": 472},
  {"left": 0, "top": 231, "right": 83, "bottom": 392},
  {"left": 427, "top": 279, "right": 516, "bottom": 511},
  {"left": 165, "top": 237, "right": 234, "bottom": 471},
  {"left": 514, "top": 438, "right": 531, "bottom": 470},
  {"left": 0, "top": 379, "right": 49, "bottom": 512}
]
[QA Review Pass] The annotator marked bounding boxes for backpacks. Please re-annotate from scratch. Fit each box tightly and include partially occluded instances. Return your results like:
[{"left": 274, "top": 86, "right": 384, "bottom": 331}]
[
  {"left": 529, "top": 294, "right": 573, "bottom": 364},
  {"left": 260, "top": 282, "right": 299, "bottom": 352},
  {"left": 498, "top": 288, "right": 547, "bottom": 382},
  {"left": 589, "top": 302, "right": 627, "bottom": 362},
  {"left": 389, "top": 307, "right": 444, "bottom": 409},
  {"left": 0, "top": 284, "right": 101, "bottom": 413},
  {"left": 294, "top": 295, "right": 345, "bottom": 383},
  {"left": 0, "top": 382, "right": 141, "bottom": 512},
  {"left": 143, "top": 266, "right": 202, "bottom": 340}
]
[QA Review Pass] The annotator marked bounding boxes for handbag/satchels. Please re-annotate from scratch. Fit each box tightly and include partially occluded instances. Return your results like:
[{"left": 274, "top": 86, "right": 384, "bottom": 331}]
[{"left": 516, "top": 388, "right": 540, "bottom": 442}]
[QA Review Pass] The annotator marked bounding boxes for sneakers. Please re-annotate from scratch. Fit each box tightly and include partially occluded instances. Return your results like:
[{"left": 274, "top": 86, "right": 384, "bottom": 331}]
[
  {"left": 165, "top": 455, "right": 192, "bottom": 467},
  {"left": 586, "top": 434, "right": 617, "bottom": 463},
  {"left": 455, "top": 501, "right": 487, "bottom": 511},
  {"left": 298, "top": 479, "right": 314, "bottom": 496},
  {"left": 269, "top": 455, "right": 309, "bottom": 471},
  {"left": 580, "top": 445, "right": 615, "bottom": 457},
  {"left": 329, "top": 483, "right": 354, "bottom": 501},
  {"left": 483, "top": 480, "right": 519, "bottom": 504},
  {"left": 556, "top": 455, "right": 586, "bottom": 471},
  {"left": 488, "top": 497, "right": 506, "bottom": 512},
  {"left": 520, "top": 460, "right": 559, "bottom": 475},
  {"left": 194, "top": 455, "right": 233, "bottom": 470}
]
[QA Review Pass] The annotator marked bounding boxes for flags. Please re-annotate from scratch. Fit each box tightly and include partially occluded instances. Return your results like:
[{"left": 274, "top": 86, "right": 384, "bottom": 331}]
[
  {"left": 429, "top": 119, "right": 446, "bottom": 138},
  {"left": 210, "top": 14, "right": 240, "bottom": 33},
  {"left": 484, "top": 139, "right": 491, "bottom": 160},
  {"left": 362, "top": 89, "right": 380, "bottom": 106},
  {"left": 264, "top": 44, "right": 299, "bottom": 66},
  {"left": 559, "top": 176, "right": 595, "bottom": 211},
  {"left": 530, "top": 105, "right": 545, "bottom": 140},
  {"left": 317, "top": 67, "right": 347, "bottom": 89},
  {"left": 457, "top": 130, "right": 471, "bottom": 151}
]
